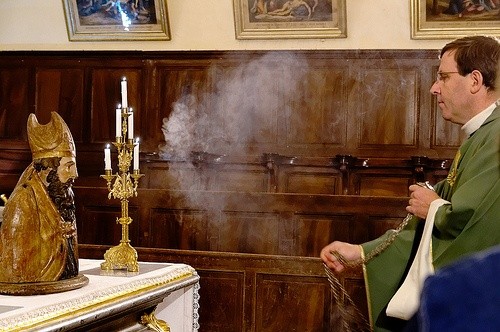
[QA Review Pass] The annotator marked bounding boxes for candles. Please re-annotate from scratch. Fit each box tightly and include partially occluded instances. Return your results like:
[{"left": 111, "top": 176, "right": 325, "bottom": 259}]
[
  {"left": 133, "top": 137, "right": 140, "bottom": 171},
  {"left": 115, "top": 103, "right": 122, "bottom": 137},
  {"left": 104, "top": 143, "right": 112, "bottom": 171},
  {"left": 120, "top": 76, "right": 128, "bottom": 109},
  {"left": 127, "top": 107, "right": 134, "bottom": 139}
]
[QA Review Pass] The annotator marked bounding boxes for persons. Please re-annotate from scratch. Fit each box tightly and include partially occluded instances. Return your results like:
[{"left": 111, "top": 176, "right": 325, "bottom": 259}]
[
  {"left": 321, "top": 35, "right": 500, "bottom": 332},
  {"left": 0, "top": 110, "right": 79, "bottom": 283}
]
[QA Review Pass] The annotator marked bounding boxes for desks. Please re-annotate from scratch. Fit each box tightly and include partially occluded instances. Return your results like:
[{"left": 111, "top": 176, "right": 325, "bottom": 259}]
[{"left": 0, "top": 258, "right": 201, "bottom": 332}]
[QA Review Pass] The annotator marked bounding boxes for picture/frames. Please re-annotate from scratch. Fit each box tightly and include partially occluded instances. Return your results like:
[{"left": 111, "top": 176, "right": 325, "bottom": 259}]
[
  {"left": 408, "top": 0, "right": 500, "bottom": 40},
  {"left": 61, "top": 0, "right": 172, "bottom": 42},
  {"left": 232, "top": 0, "right": 349, "bottom": 40}
]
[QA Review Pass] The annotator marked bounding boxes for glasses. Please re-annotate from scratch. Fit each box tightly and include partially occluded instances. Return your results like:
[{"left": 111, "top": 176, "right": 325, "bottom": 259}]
[{"left": 437, "top": 71, "right": 471, "bottom": 80}]
